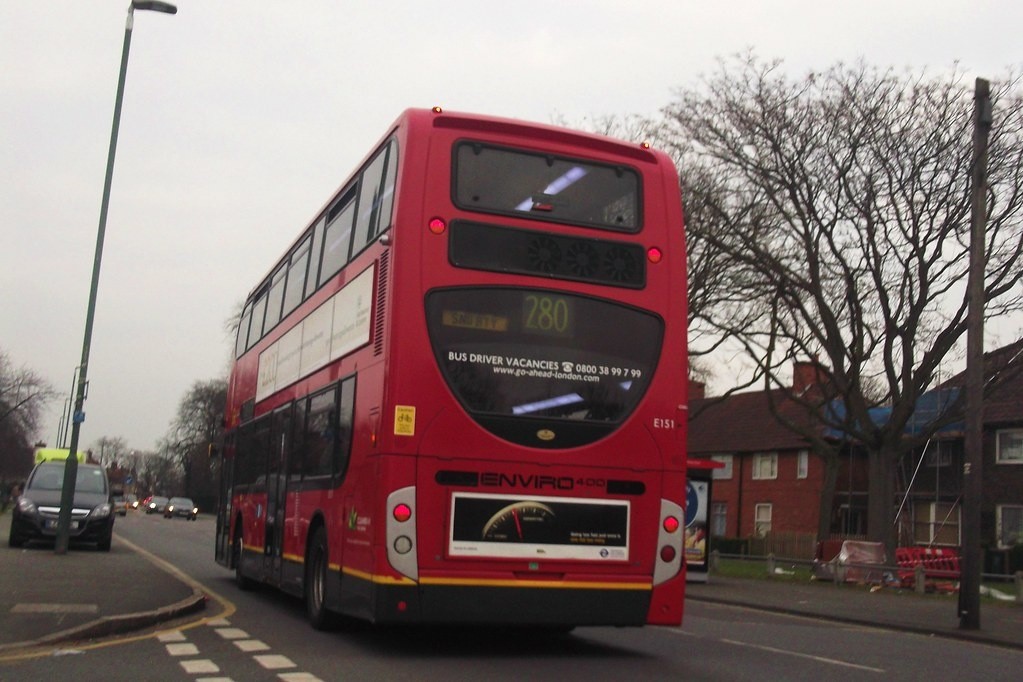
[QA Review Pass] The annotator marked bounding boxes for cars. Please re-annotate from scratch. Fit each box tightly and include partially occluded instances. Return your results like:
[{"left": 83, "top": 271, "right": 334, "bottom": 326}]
[
  {"left": 9, "top": 460, "right": 125, "bottom": 553},
  {"left": 144, "top": 495, "right": 168, "bottom": 514},
  {"left": 113, "top": 495, "right": 128, "bottom": 516},
  {"left": 126, "top": 494, "right": 138, "bottom": 510},
  {"left": 165, "top": 497, "right": 199, "bottom": 522}
]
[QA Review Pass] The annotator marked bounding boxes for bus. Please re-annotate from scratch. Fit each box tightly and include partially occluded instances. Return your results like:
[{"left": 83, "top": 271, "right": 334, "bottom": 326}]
[{"left": 209, "top": 106, "right": 688, "bottom": 631}]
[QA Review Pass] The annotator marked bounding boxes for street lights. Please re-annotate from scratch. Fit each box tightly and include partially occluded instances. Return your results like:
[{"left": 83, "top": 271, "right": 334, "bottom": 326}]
[{"left": 56, "top": 0, "right": 178, "bottom": 553}]
